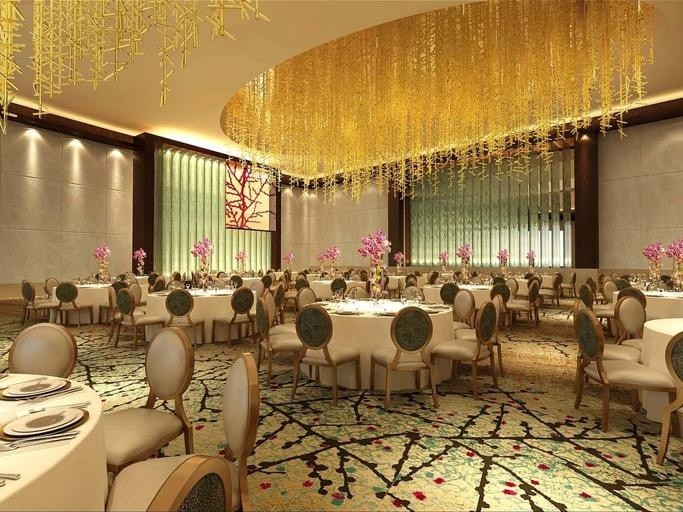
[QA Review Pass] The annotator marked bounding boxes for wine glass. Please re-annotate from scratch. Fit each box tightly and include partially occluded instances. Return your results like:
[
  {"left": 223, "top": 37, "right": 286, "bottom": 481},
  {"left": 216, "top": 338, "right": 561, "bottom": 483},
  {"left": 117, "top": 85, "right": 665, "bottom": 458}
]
[
  {"left": 632, "top": 272, "right": 682, "bottom": 296},
  {"left": 68, "top": 270, "right": 526, "bottom": 317}
]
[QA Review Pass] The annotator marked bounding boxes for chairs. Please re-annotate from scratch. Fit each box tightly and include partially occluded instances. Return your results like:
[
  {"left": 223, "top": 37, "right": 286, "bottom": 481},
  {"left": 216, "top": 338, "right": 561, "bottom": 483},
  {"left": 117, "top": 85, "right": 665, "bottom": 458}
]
[
  {"left": 106, "top": 352, "right": 261, "bottom": 511},
  {"left": 8, "top": 321, "right": 76, "bottom": 378},
  {"left": 142, "top": 455, "right": 234, "bottom": 511}
]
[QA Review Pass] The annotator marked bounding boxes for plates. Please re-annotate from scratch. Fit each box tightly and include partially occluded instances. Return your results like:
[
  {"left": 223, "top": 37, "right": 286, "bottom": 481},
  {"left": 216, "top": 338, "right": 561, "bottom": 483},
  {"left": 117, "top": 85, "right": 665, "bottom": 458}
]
[
  {"left": 2, "top": 408, "right": 83, "bottom": 437},
  {"left": 8, "top": 380, "right": 60, "bottom": 395},
  {"left": 2, "top": 379, "right": 65, "bottom": 398},
  {"left": 10, "top": 410, "right": 76, "bottom": 433}
]
